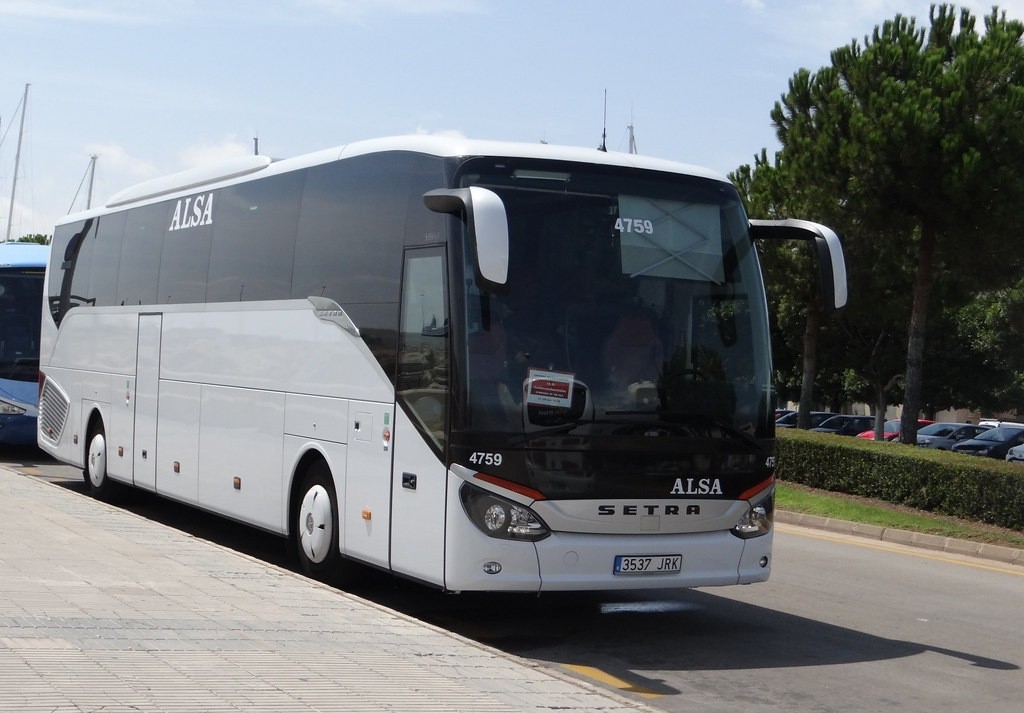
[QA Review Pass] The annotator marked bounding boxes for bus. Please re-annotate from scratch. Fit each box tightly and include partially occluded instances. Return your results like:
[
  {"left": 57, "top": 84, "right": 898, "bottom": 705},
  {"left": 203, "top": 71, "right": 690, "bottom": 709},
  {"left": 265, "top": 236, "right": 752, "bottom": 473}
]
[
  {"left": 37, "top": 135, "right": 847, "bottom": 596},
  {"left": 0, "top": 242, "right": 50, "bottom": 441}
]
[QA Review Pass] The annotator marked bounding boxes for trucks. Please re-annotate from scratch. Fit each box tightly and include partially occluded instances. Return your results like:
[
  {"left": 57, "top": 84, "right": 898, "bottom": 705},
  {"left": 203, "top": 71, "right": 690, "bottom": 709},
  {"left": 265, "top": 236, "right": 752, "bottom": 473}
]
[{"left": 975, "top": 421, "right": 1024, "bottom": 433}]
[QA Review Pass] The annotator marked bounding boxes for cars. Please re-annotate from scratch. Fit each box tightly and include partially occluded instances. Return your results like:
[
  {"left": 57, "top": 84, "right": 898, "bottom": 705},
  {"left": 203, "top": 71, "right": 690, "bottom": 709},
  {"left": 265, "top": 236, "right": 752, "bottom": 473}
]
[
  {"left": 775, "top": 409, "right": 795, "bottom": 420},
  {"left": 775, "top": 411, "right": 839, "bottom": 429},
  {"left": 1005, "top": 443, "right": 1024, "bottom": 463},
  {"left": 855, "top": 419, "right": 936, "bottom": 442},
  {"left": 890, "top": 423, "right": 992, "bottom": 451},
  {"left": 809, "top": 415, "right": 887, "bottom": 438},
  {"left": 951, "top": 427, "right": 1024, "bottom": 460}
]
